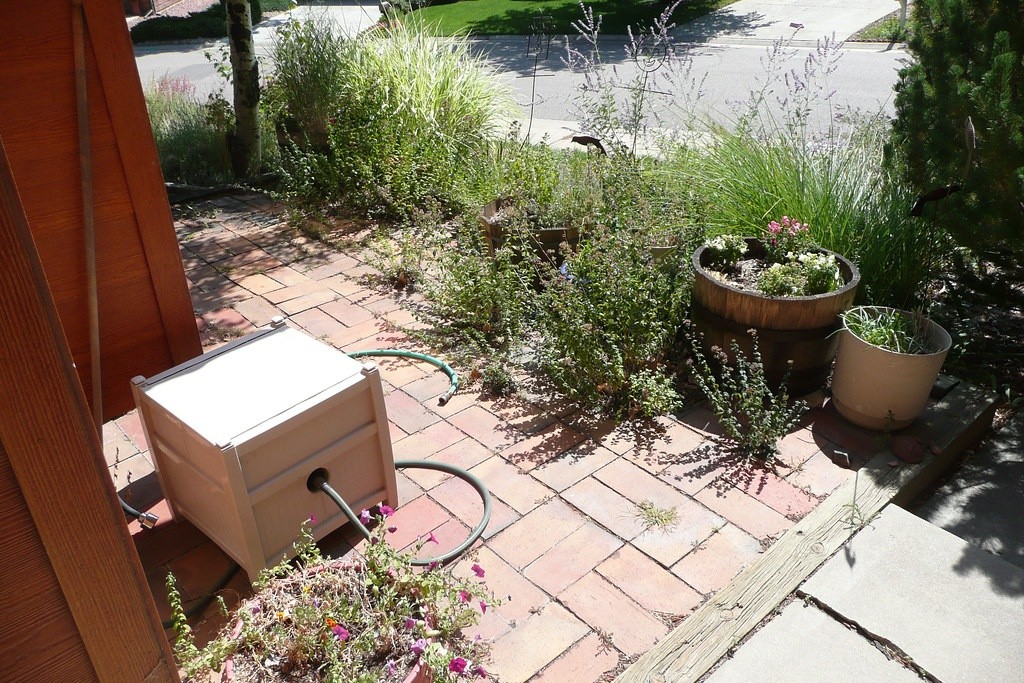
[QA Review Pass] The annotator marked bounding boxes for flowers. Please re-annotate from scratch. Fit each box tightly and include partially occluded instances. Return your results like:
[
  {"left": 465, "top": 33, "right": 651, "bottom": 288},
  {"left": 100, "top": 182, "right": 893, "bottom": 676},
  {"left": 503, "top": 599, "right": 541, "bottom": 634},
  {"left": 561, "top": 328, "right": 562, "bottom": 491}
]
[
  {"left": 154, "top": 501, "right": 491, "bottom": 683},
  {"left": 704, "top": 216, "right": 842, "bottom": 297}
]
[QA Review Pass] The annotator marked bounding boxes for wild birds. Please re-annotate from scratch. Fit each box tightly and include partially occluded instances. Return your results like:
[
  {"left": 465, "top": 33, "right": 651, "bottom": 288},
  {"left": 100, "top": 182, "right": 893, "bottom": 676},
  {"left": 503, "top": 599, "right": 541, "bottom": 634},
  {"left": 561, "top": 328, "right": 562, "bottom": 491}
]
[{"left": 571, "top": 135, "right": 607, "bottom": 156}]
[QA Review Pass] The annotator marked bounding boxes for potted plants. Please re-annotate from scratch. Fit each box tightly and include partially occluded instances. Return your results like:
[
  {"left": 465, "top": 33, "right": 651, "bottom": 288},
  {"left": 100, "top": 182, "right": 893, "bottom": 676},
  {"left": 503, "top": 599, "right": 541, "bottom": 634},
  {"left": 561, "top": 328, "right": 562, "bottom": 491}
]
[
  {"left": 480, "top": 149, "right": 601, "bottom": 295},
  {"left": 605, "top": 147, "right": 683, "bottom": 264},
  {"left": 831, "top": 306, "right": 953, "bottom": 432}
]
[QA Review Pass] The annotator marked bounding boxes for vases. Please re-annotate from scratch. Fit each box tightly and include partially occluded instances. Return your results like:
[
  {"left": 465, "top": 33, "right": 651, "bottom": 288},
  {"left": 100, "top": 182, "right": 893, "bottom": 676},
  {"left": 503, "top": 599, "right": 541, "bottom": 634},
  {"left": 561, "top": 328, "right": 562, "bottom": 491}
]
[
  {"left": 221, "top": 561, "right": 438, "bottom": 683},
  {"left": 692, "top": 237, "right": 861, "bottom": 391}
]
